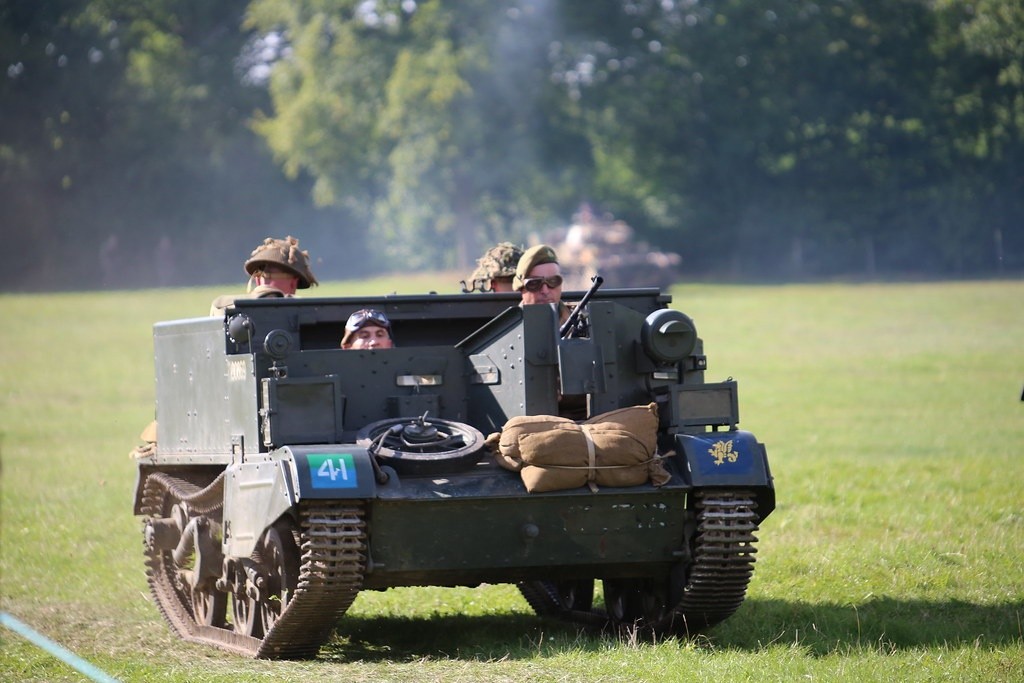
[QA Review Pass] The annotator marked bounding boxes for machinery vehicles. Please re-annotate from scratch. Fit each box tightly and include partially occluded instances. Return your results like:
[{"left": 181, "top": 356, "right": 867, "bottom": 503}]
[{"left": 131, "top": 276, "right": 778, "bottom": 664}]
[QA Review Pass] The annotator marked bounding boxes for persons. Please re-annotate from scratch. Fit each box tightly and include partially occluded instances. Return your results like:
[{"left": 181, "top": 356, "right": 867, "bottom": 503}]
[
  {"left": 209, "top": 233, "right": 318, "bottom": 320},
  {"left": 470, "top": 241, "right": 523, "bottom": 292},
  {"left": 511, "top": 244, "right": 574, "bottom": 338},
  {"left": 340, "top": 309, "right": 394, "bottom": 350}
]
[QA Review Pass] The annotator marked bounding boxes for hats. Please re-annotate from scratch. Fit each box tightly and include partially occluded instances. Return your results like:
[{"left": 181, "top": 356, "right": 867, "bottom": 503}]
[
  {"left": 512, "top": 245, "right": 557, "bottom": 291},
  {"left": 341, "top": 309, "right": 389, "bottom": 346},
  {"left": 245, "top": 239, "right": 318, "bottom": 289},
  {"left": 473, "top": 242, "right": 524, "bottom": 279}
]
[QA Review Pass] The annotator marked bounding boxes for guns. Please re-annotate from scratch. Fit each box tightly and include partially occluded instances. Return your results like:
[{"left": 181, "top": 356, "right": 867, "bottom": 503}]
[{"left": 558, "top": 275, "right": 604, "bottom": 339}]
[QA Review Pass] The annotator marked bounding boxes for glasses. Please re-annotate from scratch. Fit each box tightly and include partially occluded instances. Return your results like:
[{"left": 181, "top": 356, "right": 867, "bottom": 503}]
[{"left": 524, "top": 275, "right": 562, "bottom": 290}]
